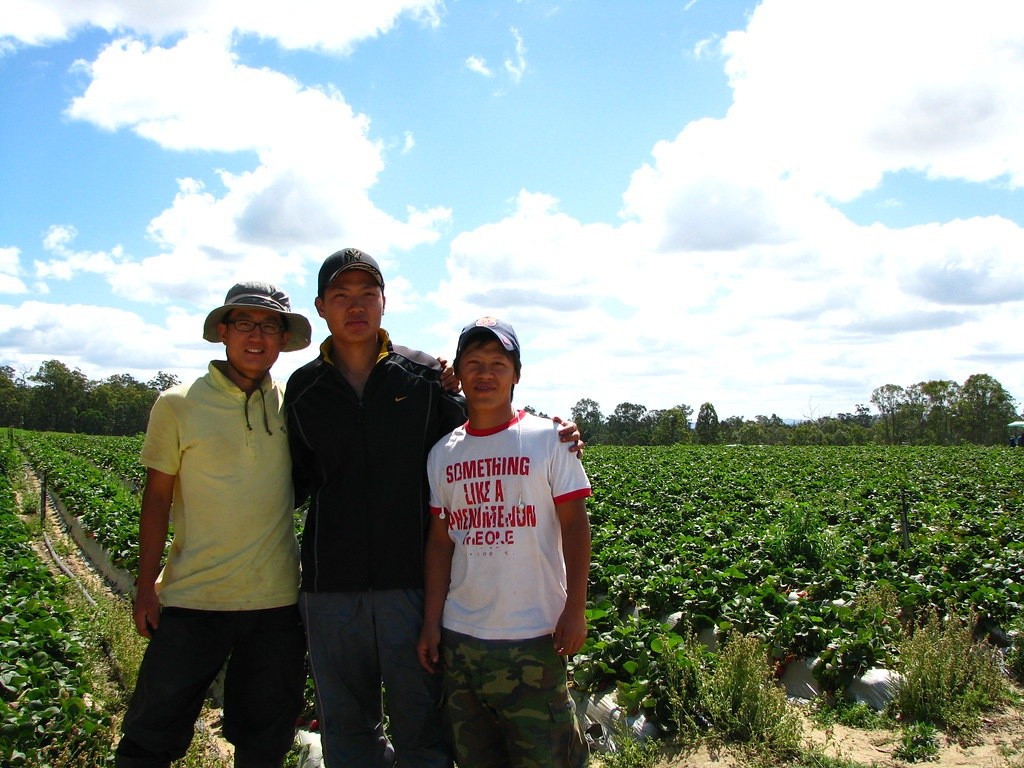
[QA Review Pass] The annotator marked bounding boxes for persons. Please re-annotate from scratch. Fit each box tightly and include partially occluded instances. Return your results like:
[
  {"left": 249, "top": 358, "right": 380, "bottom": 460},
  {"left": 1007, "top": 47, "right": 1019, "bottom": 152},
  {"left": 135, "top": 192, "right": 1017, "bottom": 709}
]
[
  {"left": 114, "top": 249, "right": 586, "bottom": 768},
  {"left": 414, "top": 317, "right": 595, "bottom": 768}
]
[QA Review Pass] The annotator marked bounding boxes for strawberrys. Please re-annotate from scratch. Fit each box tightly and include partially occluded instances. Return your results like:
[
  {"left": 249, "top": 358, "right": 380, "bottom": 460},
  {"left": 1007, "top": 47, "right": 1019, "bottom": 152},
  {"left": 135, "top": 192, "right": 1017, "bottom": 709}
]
[{"left": 295, "top": 716, "right": 320, "bottom": 732}]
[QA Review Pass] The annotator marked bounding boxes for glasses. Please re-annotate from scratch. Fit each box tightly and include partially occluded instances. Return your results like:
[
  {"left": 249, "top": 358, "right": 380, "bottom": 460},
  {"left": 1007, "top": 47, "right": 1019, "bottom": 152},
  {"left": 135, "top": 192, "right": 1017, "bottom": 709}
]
[{"left": 226, "top": 318, "right": 287, "bottom": 335}]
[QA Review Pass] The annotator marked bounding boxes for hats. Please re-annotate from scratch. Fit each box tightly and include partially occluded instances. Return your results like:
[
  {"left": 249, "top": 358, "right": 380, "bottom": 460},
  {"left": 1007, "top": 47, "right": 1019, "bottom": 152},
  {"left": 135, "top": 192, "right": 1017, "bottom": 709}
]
[
  {"left": 455, "top": 315, "right": 521, "bottom": 359},
  {"left": 318, "top": 247, "right": 384, "bottom": 289},
  {"left": 203, "top": 281, "right": 310, "bottom": 353}
]
